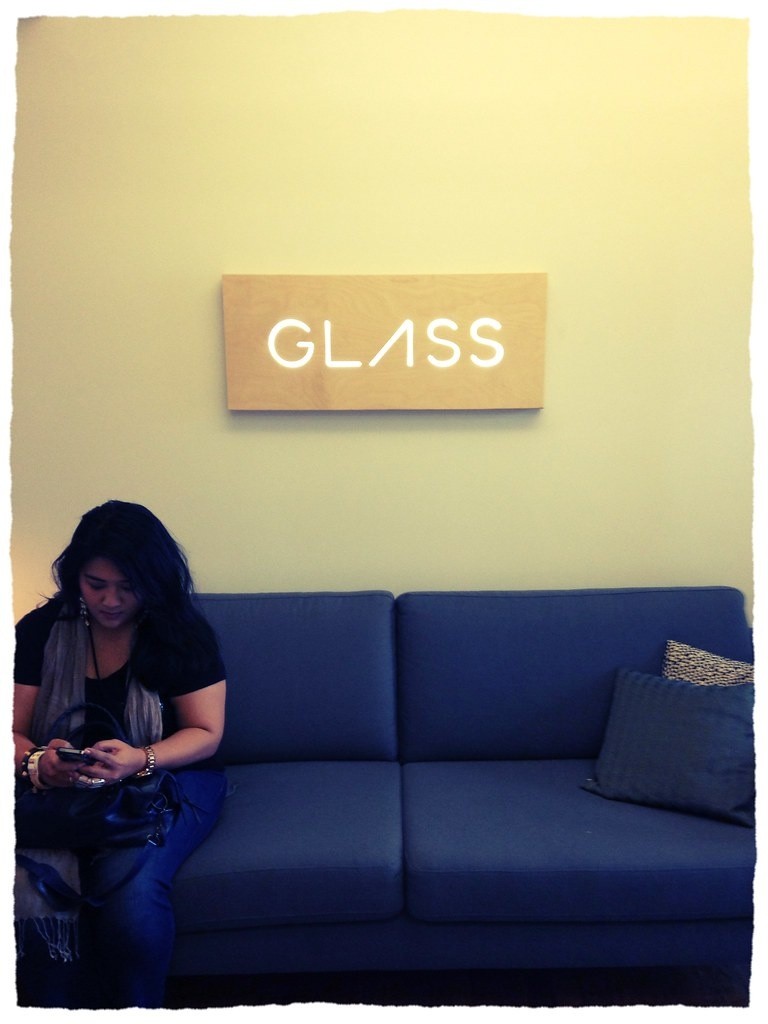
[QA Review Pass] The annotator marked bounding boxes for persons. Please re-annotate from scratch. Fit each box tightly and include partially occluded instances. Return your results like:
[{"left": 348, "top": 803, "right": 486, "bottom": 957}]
[{"left": 15, "top": 499, "right": 230, "bottom": 1008}]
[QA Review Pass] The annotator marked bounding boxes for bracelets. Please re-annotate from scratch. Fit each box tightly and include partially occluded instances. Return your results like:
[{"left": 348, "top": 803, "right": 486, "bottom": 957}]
[{"left": 19, "top": 745, "right": 55, "bottom": 794}]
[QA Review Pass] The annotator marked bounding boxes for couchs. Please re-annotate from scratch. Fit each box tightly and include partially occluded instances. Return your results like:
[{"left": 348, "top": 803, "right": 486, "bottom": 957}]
[{"left": 15, "top": 585, "right": 756, "bottom": 1009}]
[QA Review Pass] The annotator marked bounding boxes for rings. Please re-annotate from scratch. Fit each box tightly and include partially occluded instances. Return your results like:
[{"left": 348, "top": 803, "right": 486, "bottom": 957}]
[
  {"left": 69, "top": 777, "right": 73, "bottom": 783},
  {"left": 76, "top": 775, "right": 106, "bottom": 788}
]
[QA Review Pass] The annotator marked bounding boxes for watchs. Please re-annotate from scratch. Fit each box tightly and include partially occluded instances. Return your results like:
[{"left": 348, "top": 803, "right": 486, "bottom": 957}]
[{"left": 132, "top": 744, "right": 155, "bottom": 779}]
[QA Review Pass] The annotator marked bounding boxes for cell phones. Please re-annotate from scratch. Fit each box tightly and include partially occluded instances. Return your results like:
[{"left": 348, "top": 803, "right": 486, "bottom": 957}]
[{"left": 57, "top": 746, "right": 95, "bottom": 766}]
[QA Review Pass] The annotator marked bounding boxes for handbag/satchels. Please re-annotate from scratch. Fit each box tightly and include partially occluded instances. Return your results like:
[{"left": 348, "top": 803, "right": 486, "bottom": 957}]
[{"left": 16, "top": 700, "right": 179, "bottom": 907}]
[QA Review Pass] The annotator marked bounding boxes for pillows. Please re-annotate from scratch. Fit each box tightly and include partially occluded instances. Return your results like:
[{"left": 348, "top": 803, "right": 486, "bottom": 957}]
[
  {"left": 659, "top": 639, "right": 756, "bottom": 686},
  {"left": 580, "top": 665, "right": 756, "bottom": 828}
]
